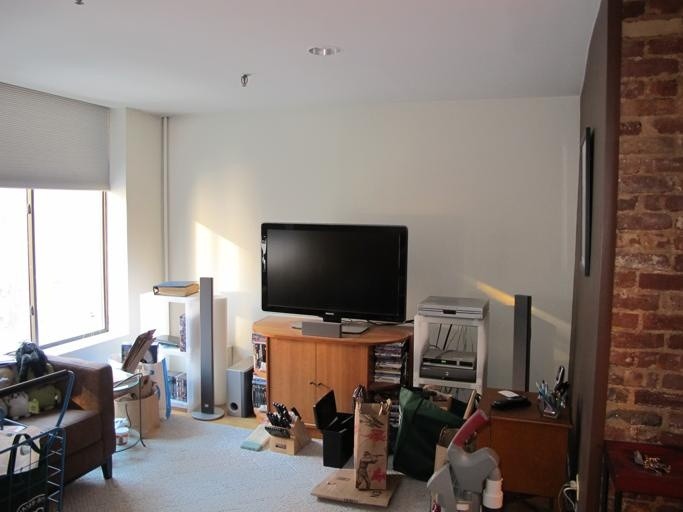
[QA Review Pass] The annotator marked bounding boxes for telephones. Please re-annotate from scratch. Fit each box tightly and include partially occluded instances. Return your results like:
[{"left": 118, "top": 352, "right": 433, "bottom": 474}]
[{"left": 550, "top": 366, "right": 565, "bottom": 397}]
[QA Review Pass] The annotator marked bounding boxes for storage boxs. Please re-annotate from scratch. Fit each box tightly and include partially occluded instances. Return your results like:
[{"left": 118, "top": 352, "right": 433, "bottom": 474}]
[
  {"left": 433, "top": 426, "right": 460, "bottom": 472},
  {"left": 354, "top": 402, "right": 390, "bottom": 490},
  {"left": 312, "top": 389, "right": 354, "bottom": 468},
  {"left": 122, "top": 342, "right": 159, "bottom": 364},
  {"left": 114, "top": 392, "right": 160, "bottom": 438}
]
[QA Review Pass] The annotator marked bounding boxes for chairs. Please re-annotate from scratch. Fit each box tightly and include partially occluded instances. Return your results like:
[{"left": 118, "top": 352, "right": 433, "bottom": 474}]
[{"left": 392, "top": 386, "right": 477, "bottom": 481}]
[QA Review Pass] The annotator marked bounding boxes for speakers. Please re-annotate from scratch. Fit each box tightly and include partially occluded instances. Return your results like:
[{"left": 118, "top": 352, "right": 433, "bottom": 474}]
[{"left": 226, "top": 359, "right": 254, "bottom": 417}]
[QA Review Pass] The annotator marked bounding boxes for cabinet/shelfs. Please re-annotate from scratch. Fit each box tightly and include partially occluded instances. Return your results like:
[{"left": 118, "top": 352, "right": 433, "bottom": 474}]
[
  {"left": 140, "top": 289, "right": 228, "bottom": 412},
  {"left": 413, "top": 315, "right": 489, "bottom": 405},
  {"left": 251, "top": 329, "right": 268, "bottom": 424},
  {"left": 266, "top": 335, "right": 367, "bottom": 440},
  {"left": 270, "top": 410, "right": 311, "bottom": 456},
  {"left": 368, "top": 334, "right": 415, "bottom": 427}
]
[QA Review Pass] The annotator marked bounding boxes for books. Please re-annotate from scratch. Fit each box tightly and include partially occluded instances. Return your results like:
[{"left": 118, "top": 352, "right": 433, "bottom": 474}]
[
  {"left": 152, "top": 280, "right": 200, "bottom": 297},
  {"left": 155, "top": 313, "right": 187, "bottom": 401},
  {"left": 251, "top": 333, "right": 267, "bottom": 411},
  {"left": 373, "top": 339, "right": 408, "bottom": 428}
]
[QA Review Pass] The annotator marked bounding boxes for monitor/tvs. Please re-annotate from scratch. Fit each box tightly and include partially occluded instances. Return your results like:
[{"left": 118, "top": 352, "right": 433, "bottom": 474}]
[{"left": 261, "top": 223, "right": 408, "bottom": 335}]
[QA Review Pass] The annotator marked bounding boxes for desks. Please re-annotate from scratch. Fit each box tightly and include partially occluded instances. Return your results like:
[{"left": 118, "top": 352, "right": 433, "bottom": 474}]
[
  {"left": 113, "top": 370, "right": 146, "bottom": 452},
  {"left": 601, "top": 441, "right": 683, "bottom": 511},
  {"left": 476, "top": 389, "right": 572, "bottom": 511}
]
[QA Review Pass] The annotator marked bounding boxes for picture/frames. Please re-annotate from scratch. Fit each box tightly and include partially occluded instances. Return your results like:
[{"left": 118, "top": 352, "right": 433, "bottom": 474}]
[{"left": 578, "top": 126, "right": 590, "bottom": 275}]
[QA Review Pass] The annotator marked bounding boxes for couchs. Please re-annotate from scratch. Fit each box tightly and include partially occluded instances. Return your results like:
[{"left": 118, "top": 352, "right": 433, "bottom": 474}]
[{"left": 0, "top": 355, "right": 116, "bottom": 511}]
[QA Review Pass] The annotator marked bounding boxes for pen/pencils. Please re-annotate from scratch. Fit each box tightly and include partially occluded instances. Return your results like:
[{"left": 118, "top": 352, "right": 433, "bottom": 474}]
[{"left": 536, "top": 380, "right": 555, "bottom": 415}]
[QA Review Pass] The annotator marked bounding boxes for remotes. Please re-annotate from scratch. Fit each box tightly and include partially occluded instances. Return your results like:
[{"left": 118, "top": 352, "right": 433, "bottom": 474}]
[{"left": 489, "top": 394, "right": 531, "bottom": 409}]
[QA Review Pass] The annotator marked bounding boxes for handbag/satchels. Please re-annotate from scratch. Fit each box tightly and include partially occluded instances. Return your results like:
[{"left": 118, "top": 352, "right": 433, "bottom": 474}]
[
  {"left": 108, "top": 358, "right": 170, "bottom": 421},
  {"left": 354, "top": 384, "right": 480, "bottom": 512},
  {"left": 0, "top": 434, "right": 47, "bottom": 512}
]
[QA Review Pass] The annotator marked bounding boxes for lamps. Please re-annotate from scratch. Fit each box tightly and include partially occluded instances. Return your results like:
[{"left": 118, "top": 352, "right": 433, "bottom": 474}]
[{"left": 192, "top": 277, "right": 225, "bottom": 421}]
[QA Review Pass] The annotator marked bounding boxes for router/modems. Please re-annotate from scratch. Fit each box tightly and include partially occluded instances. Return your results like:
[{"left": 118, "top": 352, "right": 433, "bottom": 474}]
[{"left": 422, "top": 324, "right": 477, "bottom": 367}]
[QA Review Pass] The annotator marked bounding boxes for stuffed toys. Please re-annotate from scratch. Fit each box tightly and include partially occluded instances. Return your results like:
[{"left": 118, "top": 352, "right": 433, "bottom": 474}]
[{"left": 0, "top": 342, "right": 62, "bottom": 423}]
